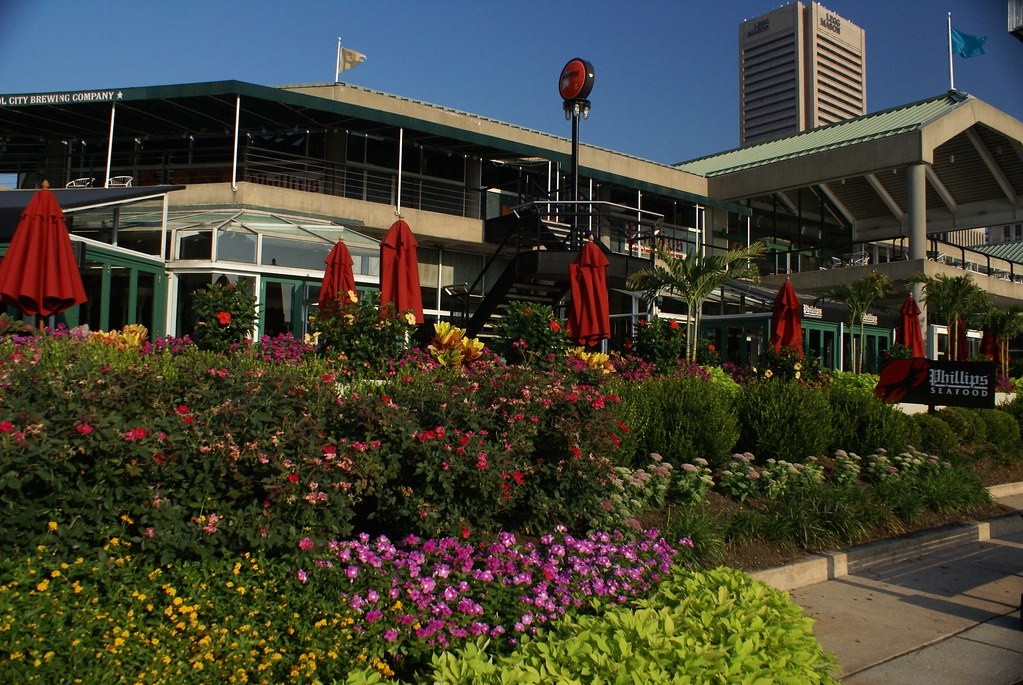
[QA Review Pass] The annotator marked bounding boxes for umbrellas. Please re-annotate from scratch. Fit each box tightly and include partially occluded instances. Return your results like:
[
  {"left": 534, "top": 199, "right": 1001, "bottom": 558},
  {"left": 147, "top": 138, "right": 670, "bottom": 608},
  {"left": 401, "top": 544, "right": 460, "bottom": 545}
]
[
  {"left": 378, "top": 215, "right": 424, "bottom": 350},
  {"left": 318, "top": 236, "right": 359, "bottom": 328},
  {"left": 895, "top": 292, "right": 926, "bottom": 358},
  {"left": 943, "top": 316, "right": 968, "bottom": 361},
  {"left": 0, "top": 180, "right": 88, "bottom": 330},
  {"left": 978, "top": 327, "right": 1011, "bottom": 371},
  {"left": 769, "top": 277, "right": 803, "bottom": 359},
  {"left": 565, "top": 235, "right": 611, "bottom": 346}
]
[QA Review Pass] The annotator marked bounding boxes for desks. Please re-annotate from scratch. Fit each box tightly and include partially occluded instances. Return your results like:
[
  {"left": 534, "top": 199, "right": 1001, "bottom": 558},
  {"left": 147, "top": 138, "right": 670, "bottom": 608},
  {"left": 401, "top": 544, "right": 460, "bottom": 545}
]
[{"left": 843, "top": 253, "right": 859, "bottom": 267}]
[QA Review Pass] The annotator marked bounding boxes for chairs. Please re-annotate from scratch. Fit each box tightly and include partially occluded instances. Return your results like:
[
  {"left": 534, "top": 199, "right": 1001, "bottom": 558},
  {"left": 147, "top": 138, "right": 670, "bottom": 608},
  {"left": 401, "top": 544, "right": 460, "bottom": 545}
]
[
  {"left": 104, "top": 175, "right": 134, "bottom": 187},
  {"left": 904, "top": 251, "right": 946, "bottom": 264},
  {"left": 832, "top": 252, "right": 871, "bottom": 269},
  {"left": 65, "top": 177, "right": 95, "bottom": 188}
]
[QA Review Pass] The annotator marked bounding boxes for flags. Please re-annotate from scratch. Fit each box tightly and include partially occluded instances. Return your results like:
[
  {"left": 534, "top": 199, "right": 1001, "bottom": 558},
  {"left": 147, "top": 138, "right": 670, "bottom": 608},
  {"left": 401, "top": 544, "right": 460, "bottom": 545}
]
[{"left": 338, "top": 46, "right": 367, "bottom": 74}]
